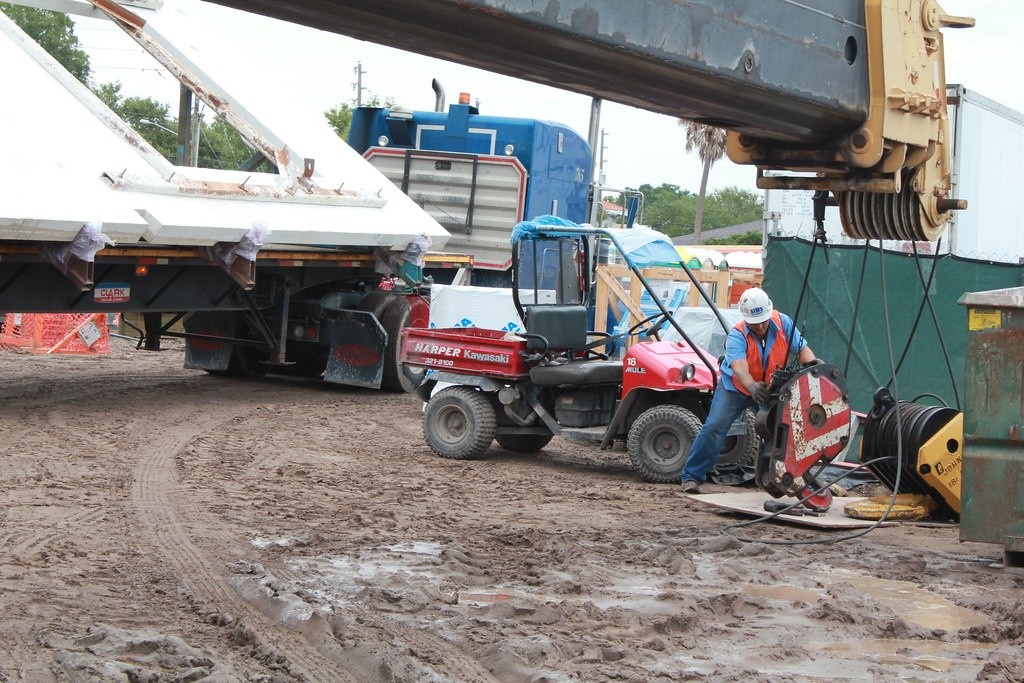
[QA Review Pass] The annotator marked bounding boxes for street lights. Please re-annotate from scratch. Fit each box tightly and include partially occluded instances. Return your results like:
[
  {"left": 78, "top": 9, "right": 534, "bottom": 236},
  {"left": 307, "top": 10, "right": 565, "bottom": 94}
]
[{"left": 141, "top": 119, "right": 199, "bottom": 168}]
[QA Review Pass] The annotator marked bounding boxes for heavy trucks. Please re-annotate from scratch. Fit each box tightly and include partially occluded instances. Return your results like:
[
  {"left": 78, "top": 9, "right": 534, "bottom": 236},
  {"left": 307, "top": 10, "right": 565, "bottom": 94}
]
[{"left": 0, "top": 78, "right": 645, "bottom": 395}]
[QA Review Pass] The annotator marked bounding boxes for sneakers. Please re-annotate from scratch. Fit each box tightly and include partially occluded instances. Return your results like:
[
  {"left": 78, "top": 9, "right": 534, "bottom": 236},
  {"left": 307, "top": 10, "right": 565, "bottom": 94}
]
[{"left": 681, "top": 480, "right": 699, "bottom": 493}]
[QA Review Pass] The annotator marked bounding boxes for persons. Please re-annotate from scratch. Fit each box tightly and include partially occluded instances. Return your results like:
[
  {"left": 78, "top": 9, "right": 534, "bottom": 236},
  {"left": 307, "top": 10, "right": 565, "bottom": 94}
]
[{"left": 681, "top": 288, "right": 825, "bottom": 492}]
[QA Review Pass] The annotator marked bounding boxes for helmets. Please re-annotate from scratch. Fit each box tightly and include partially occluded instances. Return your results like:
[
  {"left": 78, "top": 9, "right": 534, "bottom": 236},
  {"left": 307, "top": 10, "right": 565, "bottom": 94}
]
[{"left": 738, "top": 287, "right": 774, "bottom": 325}]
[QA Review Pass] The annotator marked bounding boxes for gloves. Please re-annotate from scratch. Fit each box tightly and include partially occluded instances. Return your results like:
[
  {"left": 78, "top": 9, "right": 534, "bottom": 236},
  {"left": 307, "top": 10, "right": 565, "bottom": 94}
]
[
  {"left": 802, "top": 359, "right": 825, "bottom": 366},
  {"left": 748, "top": 381, "right": 771, "bottom": 406}
]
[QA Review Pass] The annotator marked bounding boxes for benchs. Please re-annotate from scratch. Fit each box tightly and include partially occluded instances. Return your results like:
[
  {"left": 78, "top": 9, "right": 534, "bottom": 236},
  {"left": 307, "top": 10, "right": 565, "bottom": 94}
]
[{"left": 526, "top": 304, "right": 622, "bottom": 388}]
